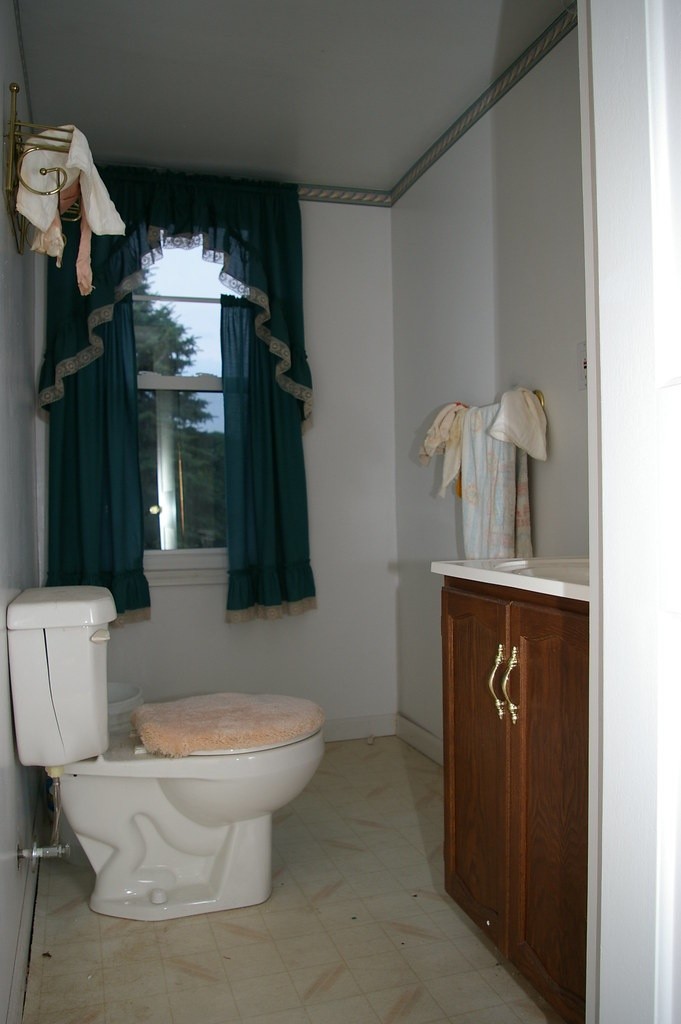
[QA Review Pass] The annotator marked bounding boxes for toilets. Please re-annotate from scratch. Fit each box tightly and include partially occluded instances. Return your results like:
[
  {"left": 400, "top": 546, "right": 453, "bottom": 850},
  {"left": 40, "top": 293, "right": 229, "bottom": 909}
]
[{"left": 5, "top": 584, "right": 326, "bottom": 924}]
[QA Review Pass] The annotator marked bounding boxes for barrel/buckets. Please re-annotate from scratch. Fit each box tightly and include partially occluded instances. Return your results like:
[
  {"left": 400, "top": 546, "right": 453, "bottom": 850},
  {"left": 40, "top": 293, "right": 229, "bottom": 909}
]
[{"left": 43, "top": 682, "right": 145, "bottom": 820}]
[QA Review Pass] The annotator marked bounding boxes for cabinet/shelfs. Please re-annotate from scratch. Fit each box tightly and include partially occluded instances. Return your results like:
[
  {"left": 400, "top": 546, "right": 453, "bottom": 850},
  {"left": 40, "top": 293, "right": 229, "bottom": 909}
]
[{"left": 440, "top": 578, "right": 590, "bottom": 1024}]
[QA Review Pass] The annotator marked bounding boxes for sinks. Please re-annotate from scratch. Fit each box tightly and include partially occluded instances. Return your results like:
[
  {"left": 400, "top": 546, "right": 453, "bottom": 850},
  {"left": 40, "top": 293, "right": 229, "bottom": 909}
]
[{"left": 507, "top": 563, "right": 589, "bottom": 586}]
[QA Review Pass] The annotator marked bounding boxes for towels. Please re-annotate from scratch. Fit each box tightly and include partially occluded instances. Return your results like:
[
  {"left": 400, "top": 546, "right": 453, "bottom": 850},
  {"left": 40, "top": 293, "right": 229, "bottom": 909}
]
[
  {"left": 458, "top": 401, "right": 534, "bottom": 561},
  {"left": 489, "top": 385, "right": 548, "bottom": 462},
  {"left": 417, "top": 400, "right": 470, "bottom": 501}
]
